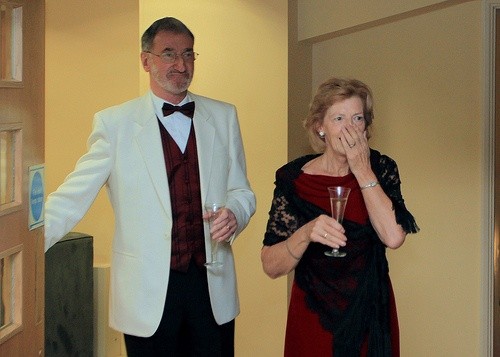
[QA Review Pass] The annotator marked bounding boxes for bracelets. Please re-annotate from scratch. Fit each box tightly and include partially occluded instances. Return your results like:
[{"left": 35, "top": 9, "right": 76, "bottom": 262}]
[
  {"left": 360, "top": 182, "right": 379, "bottom": 190},
  {"left": 285, "top": 237, "right": 300, "bottom": 260}
]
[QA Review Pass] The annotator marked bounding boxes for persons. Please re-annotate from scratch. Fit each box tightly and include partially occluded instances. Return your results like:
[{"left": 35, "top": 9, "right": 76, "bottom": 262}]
[
  {"left": 261, "top": 76, "right": 421, "bottom": 357},
  {"left": 45, "top": 17, "right": 258, "bottom": 357}
]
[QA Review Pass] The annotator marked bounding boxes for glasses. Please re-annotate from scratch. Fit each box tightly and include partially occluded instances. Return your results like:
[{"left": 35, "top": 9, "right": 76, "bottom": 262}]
[{"left": 147, "top": 50, "right": 200, "bottom": 64}]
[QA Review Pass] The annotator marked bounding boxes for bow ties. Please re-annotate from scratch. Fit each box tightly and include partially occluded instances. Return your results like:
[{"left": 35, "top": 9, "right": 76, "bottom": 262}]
[{"left": 162, "top": 100, "right": 195, "bottom": 119}]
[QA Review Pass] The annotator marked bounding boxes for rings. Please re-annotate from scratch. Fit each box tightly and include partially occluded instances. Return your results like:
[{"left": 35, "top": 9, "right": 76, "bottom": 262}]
[
  {"left": 350, "top": 144, "right": 355, "bottom": 148},
  {"left": 324, "top": 232, "right": 328, "bottom": 238}
]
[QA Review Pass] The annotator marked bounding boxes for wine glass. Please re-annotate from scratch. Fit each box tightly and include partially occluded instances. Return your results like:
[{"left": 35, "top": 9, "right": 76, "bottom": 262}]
[
  {"left": 204, "top": 204, "right": 224, "bottom": 266},
  {"left": 325, "top": 186, "right": 350, "bottom": 257}
]
